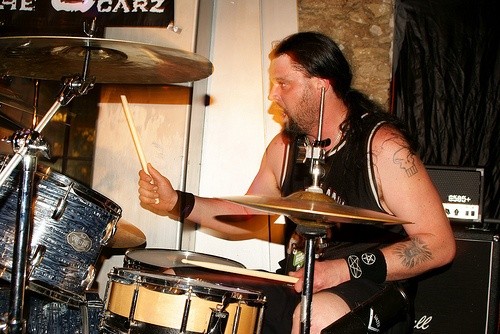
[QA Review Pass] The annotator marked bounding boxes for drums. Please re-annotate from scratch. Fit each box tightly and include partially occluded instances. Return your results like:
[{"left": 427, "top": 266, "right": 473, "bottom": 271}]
[
  {"left": 102, "top": 267, "right": 266, "bottom": 334},
  {"left": 0, "top": 153, "right": 122, "bottom": 308},
  {"left": 0, "top": 280, "right": 105, "bottom": 333},
  {"left": 124, "top": 242, "right": 248, "bottom": 280}
]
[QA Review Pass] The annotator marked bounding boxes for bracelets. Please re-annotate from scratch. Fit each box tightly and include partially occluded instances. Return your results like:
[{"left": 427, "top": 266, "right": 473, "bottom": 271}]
[
  {"left": 344, "top": 247, "right": 387, "bottom": 285},
  {"left": 168, "top": 190, "right": 195, "bottom": 218}
]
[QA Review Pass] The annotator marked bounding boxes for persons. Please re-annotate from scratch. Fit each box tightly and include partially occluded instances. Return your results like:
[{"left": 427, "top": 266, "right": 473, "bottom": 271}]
[{"left": 138, "top": 31, "right": 456, "bottom": 334}]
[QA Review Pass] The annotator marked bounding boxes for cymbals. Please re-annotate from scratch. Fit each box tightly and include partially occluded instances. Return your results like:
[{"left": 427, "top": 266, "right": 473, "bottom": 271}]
[
  {"left": 0, "top": 82, "right": 45, "bottom": 118},
  {"left": 214, "top": 187, "right": 416, "bottom": 233},
  {"left": 0, "top": 37, "right": 214, "bottom": 83},
  {"left": 105, "top": 217, "right": 146, "bottom": 249}
]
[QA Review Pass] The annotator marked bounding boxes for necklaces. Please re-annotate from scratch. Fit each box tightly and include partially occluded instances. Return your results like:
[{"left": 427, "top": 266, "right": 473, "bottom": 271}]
[{"left": 305, "top": 130, "right": 343, "bottom": 156}]
